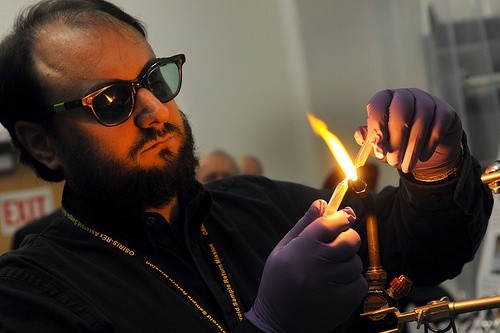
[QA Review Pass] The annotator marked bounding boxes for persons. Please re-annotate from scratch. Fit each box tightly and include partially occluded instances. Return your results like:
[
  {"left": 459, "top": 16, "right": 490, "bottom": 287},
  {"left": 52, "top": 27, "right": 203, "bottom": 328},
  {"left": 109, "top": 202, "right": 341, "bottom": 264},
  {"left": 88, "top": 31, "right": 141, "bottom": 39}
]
[
  {"left": 0, "top": 0, "right": 495, "bottom": 333},
  {"left": 235, "top": 154, "right": 263, "bottom": 175},
  {"left": 196, "top": 148, "right": 240, "bottom": 185}
]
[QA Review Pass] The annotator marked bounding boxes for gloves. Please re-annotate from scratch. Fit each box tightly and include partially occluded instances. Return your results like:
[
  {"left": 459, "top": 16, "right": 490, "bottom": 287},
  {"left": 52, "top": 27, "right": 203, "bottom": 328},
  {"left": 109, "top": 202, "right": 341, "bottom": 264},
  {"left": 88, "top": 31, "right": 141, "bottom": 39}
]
[
  {"left": 244, "top": 201, "right": 369, "bottom": 333},
  {"left": 354, "top": 88, "right": 462, "bottom": 182}
]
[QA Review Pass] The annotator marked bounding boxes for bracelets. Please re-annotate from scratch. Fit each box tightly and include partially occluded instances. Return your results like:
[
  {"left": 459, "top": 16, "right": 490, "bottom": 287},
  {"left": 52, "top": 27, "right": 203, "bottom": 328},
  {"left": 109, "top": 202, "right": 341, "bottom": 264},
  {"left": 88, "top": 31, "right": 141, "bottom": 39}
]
[{"left": 412, "top": 141, "right": 464, "bottom": 182}]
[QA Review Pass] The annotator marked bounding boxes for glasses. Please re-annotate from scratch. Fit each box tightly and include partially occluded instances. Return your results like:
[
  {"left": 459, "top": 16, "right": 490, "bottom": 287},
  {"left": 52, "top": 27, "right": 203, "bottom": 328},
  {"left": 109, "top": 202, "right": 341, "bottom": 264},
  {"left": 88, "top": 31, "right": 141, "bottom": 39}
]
[{"left": 39, "top": 54, "right": 186, "bottom": 127}]
